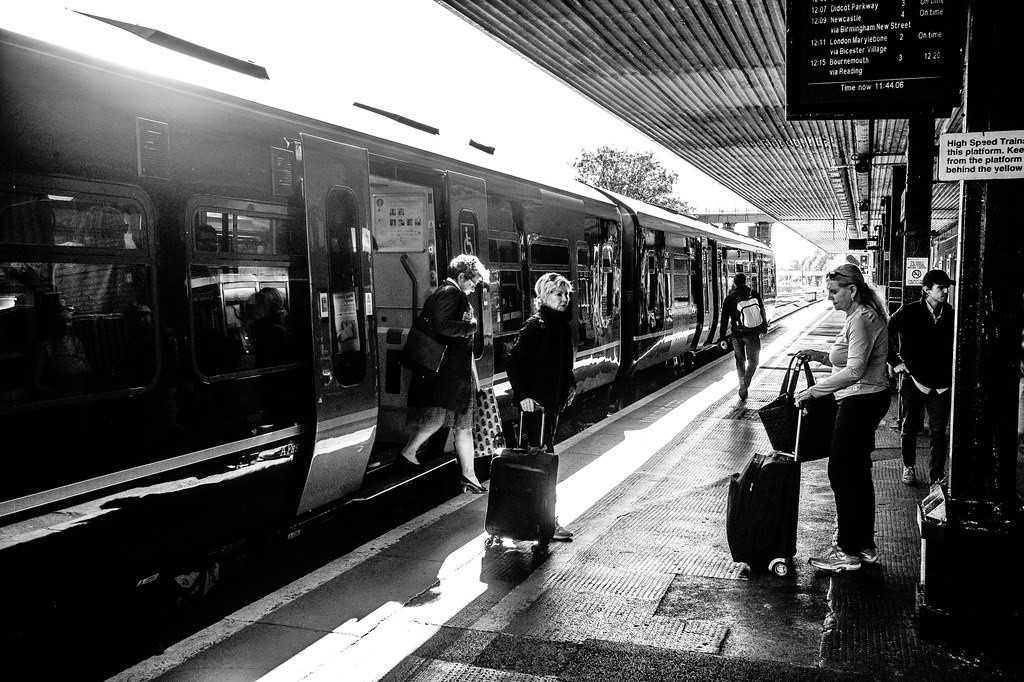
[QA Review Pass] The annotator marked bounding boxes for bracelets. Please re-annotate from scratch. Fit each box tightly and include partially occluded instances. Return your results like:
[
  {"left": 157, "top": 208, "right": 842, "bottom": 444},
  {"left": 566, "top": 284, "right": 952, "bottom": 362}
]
[{"left": 821, "top": 352, "right": 828, "bottom": 364}]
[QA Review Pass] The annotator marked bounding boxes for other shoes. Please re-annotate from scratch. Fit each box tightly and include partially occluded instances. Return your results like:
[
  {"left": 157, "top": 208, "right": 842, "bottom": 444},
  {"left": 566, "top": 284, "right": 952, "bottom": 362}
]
[
  {"left": 860, "top": 548, "right": 878, "bottom": 563},
  {"left": 902, "top": 466, "right": 916, "bottom": 484},
  {"left": 738, "top": 384, "right": 748, "bottom": 400},
  {"left": 808, "top": 550, "right": 861, "bottom": 573},
  {"left": 552, "top": 516, "right": 573, "bottom": 540}
]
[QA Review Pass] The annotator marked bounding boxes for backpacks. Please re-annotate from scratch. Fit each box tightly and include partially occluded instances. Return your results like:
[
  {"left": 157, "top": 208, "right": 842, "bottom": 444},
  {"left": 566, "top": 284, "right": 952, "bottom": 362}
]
[{"left": 733, "top": 289, "right": 763, "bottom": 328}]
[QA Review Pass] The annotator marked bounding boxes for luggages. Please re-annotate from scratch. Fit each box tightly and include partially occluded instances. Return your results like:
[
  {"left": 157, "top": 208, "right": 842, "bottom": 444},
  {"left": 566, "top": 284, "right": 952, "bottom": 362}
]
[
  {"left": 725, "top": 407, "right": 801, "bottom": 576},
  {"left": 483, "top": 404, "right": 560, "bottom": 555}
]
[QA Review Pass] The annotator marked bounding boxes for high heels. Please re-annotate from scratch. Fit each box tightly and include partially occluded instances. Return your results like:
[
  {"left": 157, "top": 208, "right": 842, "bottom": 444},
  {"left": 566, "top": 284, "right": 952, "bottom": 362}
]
[
  {"left": 395, "top": 452, "right": 428, "bottom": 471},
  {"left": 460, "top": 475, "right": 489, "bottom": 494}
]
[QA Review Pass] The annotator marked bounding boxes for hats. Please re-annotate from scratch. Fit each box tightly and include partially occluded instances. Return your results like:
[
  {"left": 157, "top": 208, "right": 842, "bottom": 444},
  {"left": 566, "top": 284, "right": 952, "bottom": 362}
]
[{"left": 922, "top": 269, "right": 956, "bottom": 288}]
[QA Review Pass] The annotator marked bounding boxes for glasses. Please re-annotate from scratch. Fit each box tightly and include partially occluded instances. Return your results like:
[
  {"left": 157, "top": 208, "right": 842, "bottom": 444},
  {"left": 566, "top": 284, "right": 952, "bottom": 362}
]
[
  {"left": 544, "top": 274, "right": 561, "bottom": 284},
  {"left": 826, "top": 271, "right": 851, "bottom": 281}
]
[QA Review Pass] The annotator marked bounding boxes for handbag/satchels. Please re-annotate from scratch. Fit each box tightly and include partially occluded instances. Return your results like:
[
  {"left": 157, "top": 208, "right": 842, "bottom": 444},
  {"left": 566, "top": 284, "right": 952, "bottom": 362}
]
[
  {"left": 471, "top": 387, "right": 507, "bottom": 458},
  {"left": 758, "top": 350, "right": 840, "bottom": 463},
  {"left": 402, "top": 316, "right": 451, "bottom": 375}
]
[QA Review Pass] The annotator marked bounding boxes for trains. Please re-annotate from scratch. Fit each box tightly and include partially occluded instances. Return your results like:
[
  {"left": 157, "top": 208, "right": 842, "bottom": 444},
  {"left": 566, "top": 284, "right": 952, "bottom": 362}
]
[{"left": 0, "top": 1, "right": 778, "bottom": 663}]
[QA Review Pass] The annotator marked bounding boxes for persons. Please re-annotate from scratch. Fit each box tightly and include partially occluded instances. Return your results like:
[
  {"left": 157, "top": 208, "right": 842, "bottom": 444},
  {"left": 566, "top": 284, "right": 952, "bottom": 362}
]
[
  {"left": 30, "top": 203, "right": 155, "bottom": 362},
  {"left": 505, "top": 272, "right": 578, "bottom": 540},
  {"left": 230, "top": 286, "right": 300, "bottom": 374},
  {"left": 189, "top": 224, "right": 225, "bottom": 309},
  {"left": 393, "top": 253, "right": 489, "bottom": 492},
  {"left": 37, "top": 297, "right": 95, "bottom": 395},
  {"left": 720, "top": 273, "right": 768, "bottom": 400},
  {"left": 887, "top": 270, "right": 957, "bottom": 487},
  {"left": 786, "top": 264, "right": 892, "bottom": 572}
]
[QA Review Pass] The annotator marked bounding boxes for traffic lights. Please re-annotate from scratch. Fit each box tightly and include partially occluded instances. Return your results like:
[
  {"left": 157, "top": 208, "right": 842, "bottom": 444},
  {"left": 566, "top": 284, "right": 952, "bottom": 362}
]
[
  {"left": 861, "top": 256, "right": 866, "bottom": 261},
  {"left": 865, "top": 266, "right": 868, "bottom": 269}
]
